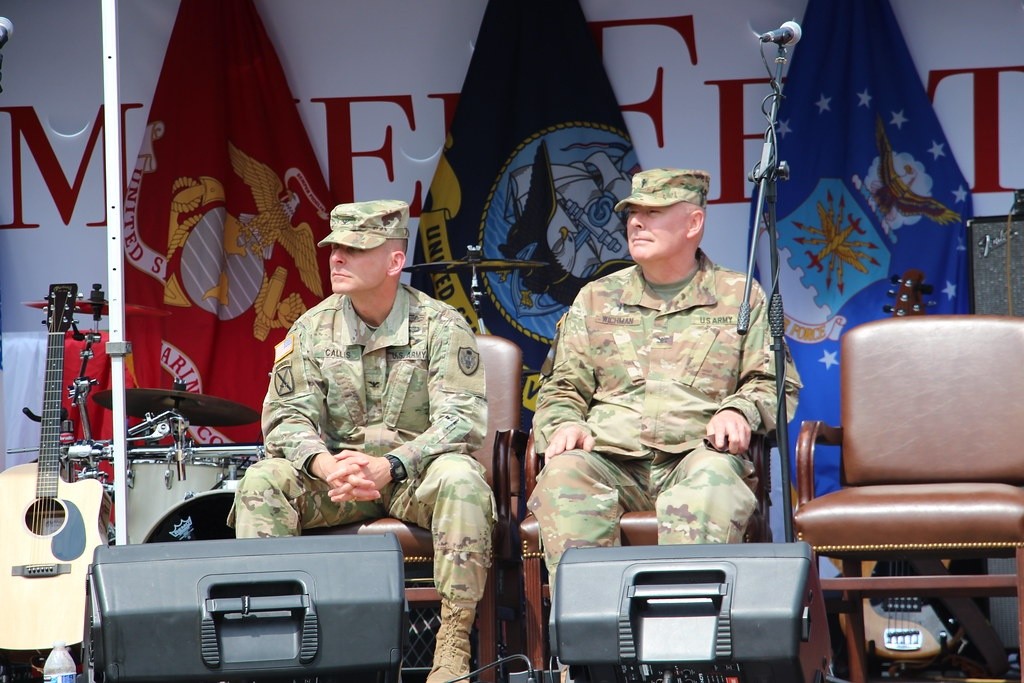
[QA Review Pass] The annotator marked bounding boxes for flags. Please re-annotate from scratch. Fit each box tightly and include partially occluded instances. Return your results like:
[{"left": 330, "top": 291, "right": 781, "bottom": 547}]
[
  {"left": 747, "top": 1, "right": 975, "bottom": 584},
  {"left": 408, "top": 1, "right": 643, "bottom": 446},
  {"left": 66, "top": 0, "right": 336, "bottom": 532}
]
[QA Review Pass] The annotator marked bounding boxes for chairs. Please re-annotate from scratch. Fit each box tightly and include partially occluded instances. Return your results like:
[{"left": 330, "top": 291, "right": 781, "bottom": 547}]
[
  {"left": 304, "top": 333, "right": 523, "bottom": 683},
  {"left": 517, "top": 424, "right": 772, "bottom": 670},
  {"left": 793, "top": 315, "right": 1024, "bottom": 682}
]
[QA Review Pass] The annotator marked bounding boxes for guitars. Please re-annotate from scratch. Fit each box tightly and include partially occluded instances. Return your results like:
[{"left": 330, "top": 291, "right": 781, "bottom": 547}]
[
  {"left": 0, "top": 282, "right": 112, "bottom": 645},
  {"left": 834, "top": 268, "right": 968, "bottom": 662}
]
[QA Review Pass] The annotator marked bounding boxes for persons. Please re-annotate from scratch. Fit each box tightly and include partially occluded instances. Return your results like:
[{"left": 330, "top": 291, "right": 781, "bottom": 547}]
[
  {"left": 228, "top": 200, "right": 497, "bottom": 683},
  {"left": 527, "top": 168, "right": 803, "bottom": 683}
]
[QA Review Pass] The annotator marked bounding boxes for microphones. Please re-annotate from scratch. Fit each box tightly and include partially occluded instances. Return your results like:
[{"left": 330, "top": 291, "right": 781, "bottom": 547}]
[
  {"left": 759, "top": 20, "right": 801, "bottom": 47},
  {"left": 0, "top": 17, "right": 14, "bottom": 49}
]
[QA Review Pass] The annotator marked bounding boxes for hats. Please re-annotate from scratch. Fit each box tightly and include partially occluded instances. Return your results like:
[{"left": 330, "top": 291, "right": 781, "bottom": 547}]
[
  {"left": 613, "top": 168, "right": 711, "bottom": 213},
  {"left": 317, "top": 200, "right": 410, "bottom": 252}
]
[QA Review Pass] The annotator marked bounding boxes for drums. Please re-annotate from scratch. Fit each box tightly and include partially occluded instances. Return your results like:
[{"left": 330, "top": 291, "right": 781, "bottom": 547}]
[
  {"left": 127, "top": 448, "right": 258, "bottom": 543},
  {"left": 142, "top": 489, "right": 238, "bottom": 543}
]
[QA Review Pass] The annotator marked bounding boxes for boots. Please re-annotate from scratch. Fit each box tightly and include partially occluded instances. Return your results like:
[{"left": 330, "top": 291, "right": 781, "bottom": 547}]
[{"left": 426, "top": 599, "right": 476, "bottom": 683}]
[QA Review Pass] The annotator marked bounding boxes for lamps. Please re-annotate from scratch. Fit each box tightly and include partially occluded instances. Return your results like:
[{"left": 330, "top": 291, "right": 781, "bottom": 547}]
[
  {"left": 549, "top": 542, "right": 814, "bottom": 683},
  {"left": 81, "top": 533, "right": 408, "bottom": 683}
]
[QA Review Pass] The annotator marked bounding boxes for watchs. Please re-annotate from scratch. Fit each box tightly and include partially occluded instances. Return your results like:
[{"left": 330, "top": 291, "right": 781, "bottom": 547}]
[{"left": 384, "top": 454, "right": 408, "bottom": 481}]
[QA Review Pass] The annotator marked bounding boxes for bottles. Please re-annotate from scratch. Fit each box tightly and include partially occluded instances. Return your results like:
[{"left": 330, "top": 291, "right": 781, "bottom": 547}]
[{"left": 43, "top": 641, "right": 76, "bottom": 683}]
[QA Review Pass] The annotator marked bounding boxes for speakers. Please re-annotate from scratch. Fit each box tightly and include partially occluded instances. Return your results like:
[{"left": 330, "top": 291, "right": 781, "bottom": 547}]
[
  {"left": 549, "top": 541, "right": 832, "bottom": 683},
  {"left": 82, "top": 531, "right": 409, "bottom": 683}
]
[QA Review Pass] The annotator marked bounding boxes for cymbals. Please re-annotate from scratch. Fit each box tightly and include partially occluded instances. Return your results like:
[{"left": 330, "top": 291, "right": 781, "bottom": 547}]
[
  {"left": 399, "top": 256, "right": 551, "bottom": 273},
  {"left": 90, "top": 387, "right": 260, "bottom": 427},
  {"left": 21, "top": 298, "right": 172, "bottom": 317}
]
